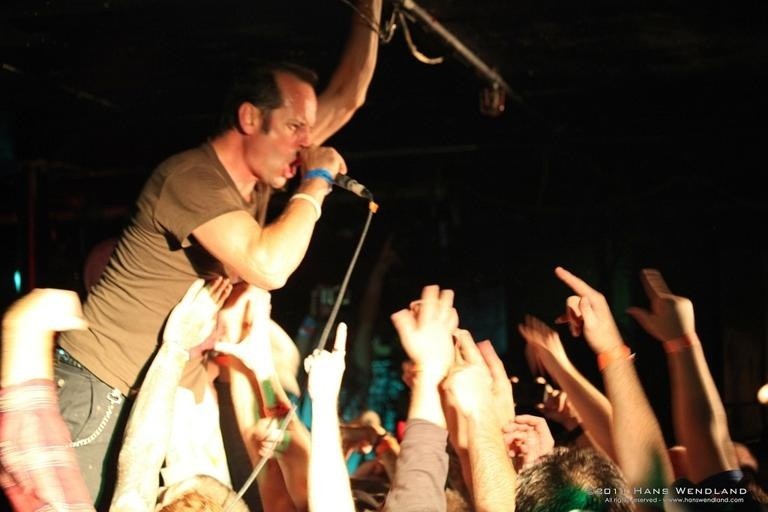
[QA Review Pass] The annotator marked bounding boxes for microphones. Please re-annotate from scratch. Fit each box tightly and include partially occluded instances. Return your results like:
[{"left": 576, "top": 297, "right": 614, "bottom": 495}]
[{"left": 333, "top": 172, "right": 373, "bottom": 199}]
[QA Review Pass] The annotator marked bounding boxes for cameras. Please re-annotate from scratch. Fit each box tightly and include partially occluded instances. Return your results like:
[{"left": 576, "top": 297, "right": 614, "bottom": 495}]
[{"left": 319, "top": 285, "right": 351, "bottom": 305}]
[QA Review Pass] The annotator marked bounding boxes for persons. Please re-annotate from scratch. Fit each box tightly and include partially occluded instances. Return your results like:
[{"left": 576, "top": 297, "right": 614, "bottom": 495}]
[
  {"left": 0, "top": 244, "right": 768, "bottom": 512},
  {"left": 40, "top": 0, "right": 381, "bottom": 512}
]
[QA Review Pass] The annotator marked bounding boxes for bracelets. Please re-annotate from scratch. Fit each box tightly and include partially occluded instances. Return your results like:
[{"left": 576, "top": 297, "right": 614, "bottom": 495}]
[
  {"left": 291, "top": 194, "right": 323, "bottom": 221},
  {"left": 301, "top": 168, "right": 335, "bottom": 196}
]
